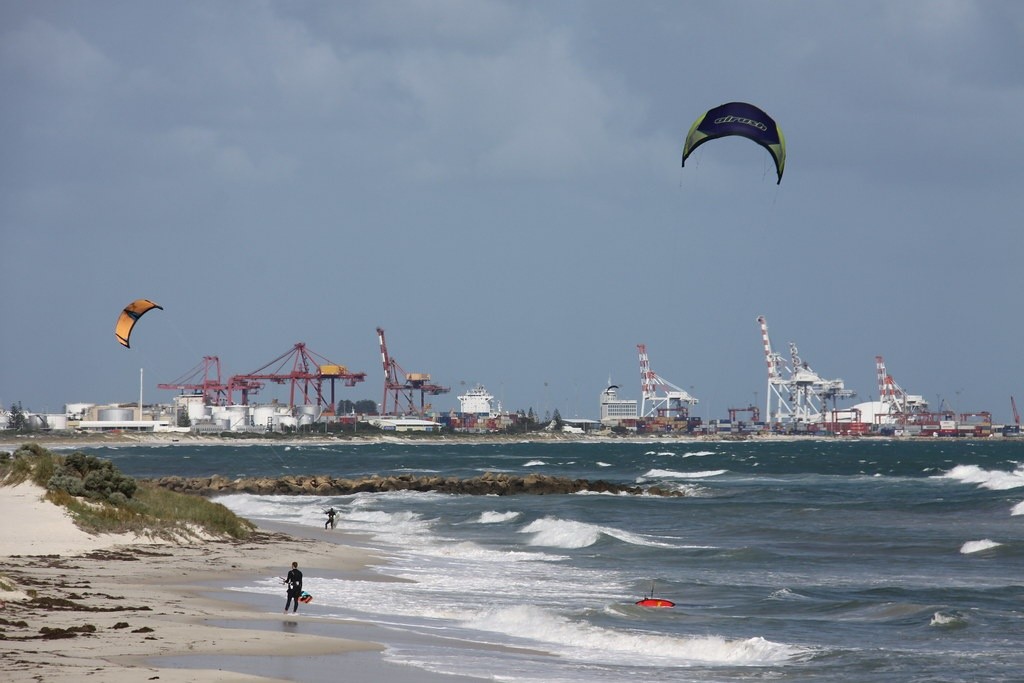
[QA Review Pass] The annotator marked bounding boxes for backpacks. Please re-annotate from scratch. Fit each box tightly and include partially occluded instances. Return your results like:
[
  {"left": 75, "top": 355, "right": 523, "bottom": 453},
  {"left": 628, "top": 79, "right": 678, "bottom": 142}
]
[{"left": 290, "top": 573, "right": 301, "bottom": 590}]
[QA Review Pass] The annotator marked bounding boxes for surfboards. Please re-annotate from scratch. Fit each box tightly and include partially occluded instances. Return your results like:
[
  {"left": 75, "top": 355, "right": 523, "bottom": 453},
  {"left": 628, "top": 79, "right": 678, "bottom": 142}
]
[
  {"left": 298, "top": 591, "right": 313, "bottom": 604},
  {"left": 635, "top": 596, "right": 678, "bottom": 608},
  {"left": 332, "top": 510, "right": 342, "bottom": 532}
]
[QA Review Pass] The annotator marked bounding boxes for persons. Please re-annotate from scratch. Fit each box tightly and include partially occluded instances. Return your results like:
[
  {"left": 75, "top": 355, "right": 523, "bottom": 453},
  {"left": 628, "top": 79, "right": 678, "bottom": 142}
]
[
  {"left": 284, "top": 562, "right": 302, "bottom": 614},
  {"left": 325, "top": 508, "right": 336, "bottom": 530}
]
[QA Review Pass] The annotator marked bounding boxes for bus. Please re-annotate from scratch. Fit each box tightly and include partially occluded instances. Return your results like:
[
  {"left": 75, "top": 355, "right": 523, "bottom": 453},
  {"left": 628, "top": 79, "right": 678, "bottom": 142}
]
[{"left": 561, "top": 419, "right": 603, "bottom": 436}]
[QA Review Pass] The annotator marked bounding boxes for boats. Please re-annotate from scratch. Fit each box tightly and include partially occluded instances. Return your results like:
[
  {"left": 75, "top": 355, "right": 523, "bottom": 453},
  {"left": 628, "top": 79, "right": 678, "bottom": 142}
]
[{"left": 635, "top": 579, "right": 675, "bottom": 609}]
